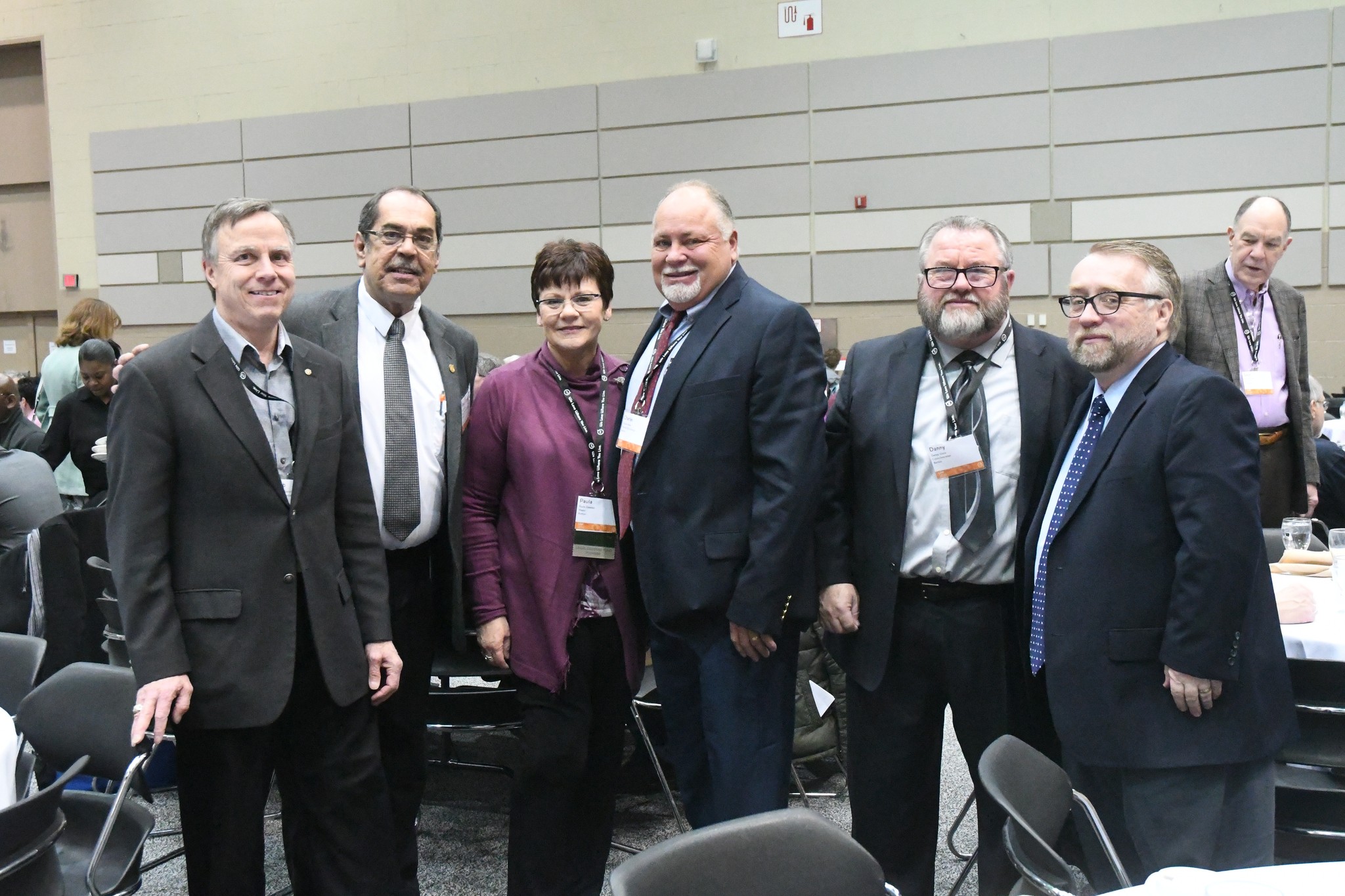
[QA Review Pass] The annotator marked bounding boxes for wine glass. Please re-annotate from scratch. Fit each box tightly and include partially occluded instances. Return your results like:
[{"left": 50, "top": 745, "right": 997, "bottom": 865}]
[{"left": 1331, "top": 555, "right": 1345, "bottom": 614}]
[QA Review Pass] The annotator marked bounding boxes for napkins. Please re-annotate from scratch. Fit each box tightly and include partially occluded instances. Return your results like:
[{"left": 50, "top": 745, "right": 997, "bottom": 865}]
[
  {"left": 1268, "top": 563, "right": 1333, "bottom": 578},
  {"left": 1279, "top": 548, "right": 1333, "bottom": 566}
]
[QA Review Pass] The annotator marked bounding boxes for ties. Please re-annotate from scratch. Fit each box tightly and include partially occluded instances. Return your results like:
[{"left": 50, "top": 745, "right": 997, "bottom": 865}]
[
  {"left": 1029, "top": 394, "right": 1109, "bottom": 678},
  {"left": 617, "top": 310, "right": 685, "bottom": 539},
  {"left": 382, "top": 318, "right": 421, "bottom": 542},
  {"left": 947, "top": 349, "right": 997, "bottom": 553}
]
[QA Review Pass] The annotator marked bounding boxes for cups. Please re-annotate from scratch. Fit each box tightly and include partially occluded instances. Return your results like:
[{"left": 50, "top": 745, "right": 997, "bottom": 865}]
[
  {"left": 1328, "top": 528, "right": 1345, "bottom": 560},
  {"left": 1281, "top": 517, "right": 1312, "bottom": 550},
  {"left": 1339, "top": 406, "right": 1345, "bottom": 420}
]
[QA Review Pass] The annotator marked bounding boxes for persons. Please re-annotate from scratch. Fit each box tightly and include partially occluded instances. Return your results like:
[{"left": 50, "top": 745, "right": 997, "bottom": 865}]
[
  {"left": 474, "top": 354, "right": 502, "bottom": 395},
  {"left": 106, "top": 200, "right": 405, "bottom": 896},
  {"left": 112, "top": 186, "right": 481, "bottom": 896},
  {"left": 822, "top": 348, "right": 841, "bottom": 385},
  {"left": 809, "top": 214, "right": 1093, "bottom": 896},
  {"left": 605, "top": 181, "right": 831, "bottom": 830},
  {"left": 1017, "top": 240, "right": 1298, "bottom": 894},
  {"left": 460, "top": 240, "right": 650, "bottom": 896},
  {"left": 0, "top": 297, "right": 122, "bottom": 553},
  {"left": 1308, "top": 376, "right": 1345, "bottom": 531},
  {"left": 1172, "top": 196, "right": 1321, "bottom": 529}
]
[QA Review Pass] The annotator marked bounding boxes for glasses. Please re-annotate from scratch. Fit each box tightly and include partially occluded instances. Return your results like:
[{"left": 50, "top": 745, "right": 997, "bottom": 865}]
[
  {"left": 1058, "top": 292, "right": 1162, "bottom": 318},
  {"left": 1314, "top": 400, "right": 1329, "bottom": 411},
  {"left": 922, "top": 266, "right": 1009, "bottom": 289},
  {"left": 536, "top": 293, "right": 603, "bottom": 316},
  {"left": 361, "top": 230, "right": 439, "bottom": 252}
]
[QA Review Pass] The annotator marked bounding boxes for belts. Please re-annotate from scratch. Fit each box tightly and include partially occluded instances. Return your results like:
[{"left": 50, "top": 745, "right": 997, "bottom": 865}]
[
  {"left": 1259, "top": 426, "right": 1291, "bottom": 446},
  {"left": 900, "top": 583, "right": 1006, "bottom": 602}
]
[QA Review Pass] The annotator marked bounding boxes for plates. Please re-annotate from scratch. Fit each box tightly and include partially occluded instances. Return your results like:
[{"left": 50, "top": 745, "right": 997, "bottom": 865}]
[
  {"left": 91, "top": 453, "right": 107, "bottom": 463},
  {"left": 95, "top": 436, "right": 107, "bottom": 445},
  {"left": 91, "top": 445, "right": 107, "bottom": 453}
]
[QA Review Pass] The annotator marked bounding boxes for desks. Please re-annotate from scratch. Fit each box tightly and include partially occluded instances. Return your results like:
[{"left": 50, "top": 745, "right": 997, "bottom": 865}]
[
  {"left": 1267, "top": 550, "right": 1345, "bottom": 836},
  {"left": 1095, "top": 861, "right": 1345, "bottom": 896}
]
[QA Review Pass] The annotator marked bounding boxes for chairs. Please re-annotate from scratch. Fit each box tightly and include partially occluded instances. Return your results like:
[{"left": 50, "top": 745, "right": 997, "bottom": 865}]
[{"left": 0, "top": 504, "right": 1345, "bottom": 896}]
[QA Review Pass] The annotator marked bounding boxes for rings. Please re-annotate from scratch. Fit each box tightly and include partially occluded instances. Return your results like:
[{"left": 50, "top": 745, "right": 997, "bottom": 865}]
[
  {"left": 752, "top": 636, "right": 758, "bottom": 641},
  {"left": 133, "top": 704, "right": 142, "bottom": 714},
  {"left": 1199, "top": 688, "right": 1212, "bottom": 693},
  {"left": 485, "top": 655, "right": 493, "bottom": 661}
]
